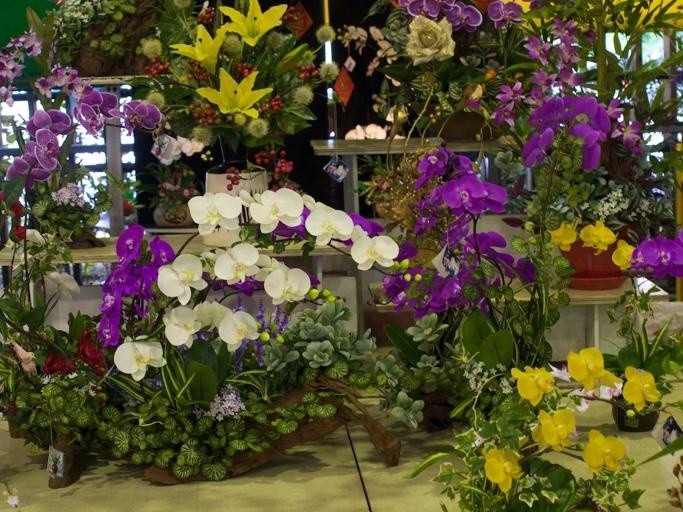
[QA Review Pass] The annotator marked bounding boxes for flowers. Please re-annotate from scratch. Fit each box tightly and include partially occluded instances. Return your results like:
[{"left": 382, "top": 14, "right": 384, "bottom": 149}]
[{"left": 1, "top": 1, "right": 683, "bottom": 512}]
